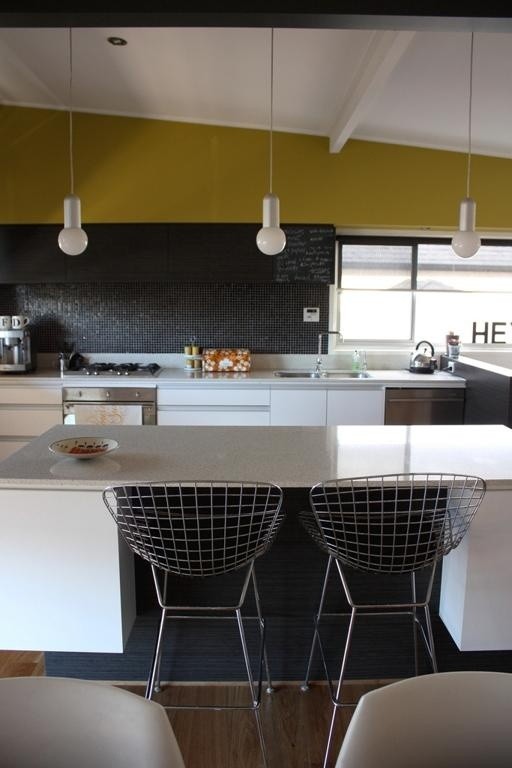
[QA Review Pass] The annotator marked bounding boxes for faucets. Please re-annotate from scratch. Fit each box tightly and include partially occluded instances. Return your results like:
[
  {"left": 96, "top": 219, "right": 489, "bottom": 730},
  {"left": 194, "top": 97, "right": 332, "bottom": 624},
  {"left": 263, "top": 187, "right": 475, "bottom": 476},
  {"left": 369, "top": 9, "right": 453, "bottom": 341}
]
[{"left": 314, "top": 332, "right": 343, "bottom": 370}]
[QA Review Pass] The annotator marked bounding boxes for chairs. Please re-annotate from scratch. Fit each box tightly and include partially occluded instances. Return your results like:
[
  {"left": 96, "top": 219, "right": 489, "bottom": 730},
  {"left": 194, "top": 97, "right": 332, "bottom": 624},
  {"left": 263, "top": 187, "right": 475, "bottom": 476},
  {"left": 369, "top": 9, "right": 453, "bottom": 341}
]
[
  {"left": 101, "top": 480, "right": 282, "bottom": 768},
  {"left": 0, "top": 676, "right": 183, "bottom": 766},
  {"left": 301, "top": 474, "right": 487, "bottom": 768},
  {"left": 333, "top": 670, "right": 512, "bottom": 768}
]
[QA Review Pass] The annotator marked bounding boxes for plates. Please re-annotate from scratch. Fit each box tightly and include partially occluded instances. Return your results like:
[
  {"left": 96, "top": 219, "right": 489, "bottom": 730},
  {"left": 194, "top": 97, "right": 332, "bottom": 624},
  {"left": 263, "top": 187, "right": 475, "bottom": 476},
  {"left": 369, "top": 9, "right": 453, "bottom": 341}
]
[{"left": 48, "top": 437, "right": 119, "bottom": 460}]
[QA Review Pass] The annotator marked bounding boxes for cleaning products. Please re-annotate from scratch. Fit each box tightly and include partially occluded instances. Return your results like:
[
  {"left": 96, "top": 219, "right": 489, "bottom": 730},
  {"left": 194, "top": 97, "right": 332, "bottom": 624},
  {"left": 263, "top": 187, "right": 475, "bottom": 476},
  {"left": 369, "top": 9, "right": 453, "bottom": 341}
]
[{"left": 351, "top": 350, "right": 361, "bottom": 370}]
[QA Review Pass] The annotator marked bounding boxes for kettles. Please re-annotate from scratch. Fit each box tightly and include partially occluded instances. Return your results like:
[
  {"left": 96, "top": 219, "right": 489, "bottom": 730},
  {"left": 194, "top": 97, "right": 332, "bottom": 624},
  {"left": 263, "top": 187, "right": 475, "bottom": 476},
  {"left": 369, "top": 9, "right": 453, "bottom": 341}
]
[{"left": 408, "top": 340, "right": 437, "bottom": 374}]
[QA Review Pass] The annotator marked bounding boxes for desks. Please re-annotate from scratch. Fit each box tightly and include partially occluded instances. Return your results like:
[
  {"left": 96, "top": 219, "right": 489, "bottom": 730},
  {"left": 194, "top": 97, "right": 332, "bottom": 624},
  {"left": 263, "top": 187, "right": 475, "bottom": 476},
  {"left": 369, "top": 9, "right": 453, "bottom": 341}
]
[{"left": 0, "top": 425, "right": 512, "bottom": 653}]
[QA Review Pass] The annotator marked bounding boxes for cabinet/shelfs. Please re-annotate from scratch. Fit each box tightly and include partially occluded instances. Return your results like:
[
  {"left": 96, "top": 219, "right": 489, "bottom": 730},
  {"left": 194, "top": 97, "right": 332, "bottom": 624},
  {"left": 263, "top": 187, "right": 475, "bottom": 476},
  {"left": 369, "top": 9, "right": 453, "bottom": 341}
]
[
  {"left": 0, "top": 366, "right": 62, "bottom": 441},
  {"left": 270, "top": 370, "right": 385, "bottom": 425},
  {"left": 1, "top": 224, "right": 65, "bottom": 282},
  {"left": 156, "top": 368, "right": 271, "bottom": 425},
  {"left": 169, "top": 224, "right": 272, "bottom": 283},
  {"left": 367, "top": 369, "right": 465, "bottom": 425},
  {"left": 64, "top": 224, "right": 169, "bottom": 283}
]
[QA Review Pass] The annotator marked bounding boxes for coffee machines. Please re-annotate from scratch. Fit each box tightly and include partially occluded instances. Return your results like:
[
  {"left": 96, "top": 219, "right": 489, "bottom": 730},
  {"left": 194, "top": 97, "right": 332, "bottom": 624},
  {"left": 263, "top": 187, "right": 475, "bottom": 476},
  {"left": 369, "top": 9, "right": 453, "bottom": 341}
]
[{"left": 0, "top": 330, "right": 31, "bottom": 373}]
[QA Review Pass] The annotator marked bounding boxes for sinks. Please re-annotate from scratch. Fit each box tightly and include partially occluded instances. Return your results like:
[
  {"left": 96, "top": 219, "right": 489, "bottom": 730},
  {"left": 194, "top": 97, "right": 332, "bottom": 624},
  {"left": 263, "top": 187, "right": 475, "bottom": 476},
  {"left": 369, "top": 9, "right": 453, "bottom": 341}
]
[{"left": 274, "top": 372, "right": 371, "bottom": 379}]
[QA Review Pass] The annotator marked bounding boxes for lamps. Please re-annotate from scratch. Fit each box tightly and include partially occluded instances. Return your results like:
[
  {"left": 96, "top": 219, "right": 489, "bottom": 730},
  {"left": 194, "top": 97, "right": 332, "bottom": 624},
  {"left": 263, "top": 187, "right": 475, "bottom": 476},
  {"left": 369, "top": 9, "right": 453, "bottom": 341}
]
[
  {"left": 58, "top": 28, "right": 87, "bottom": 255},
  {"left": 451, "top": 31, "right": 480, "bottom": 258},
  {"left": 257, "top": 27, "right": 286, "bottom": 254}
]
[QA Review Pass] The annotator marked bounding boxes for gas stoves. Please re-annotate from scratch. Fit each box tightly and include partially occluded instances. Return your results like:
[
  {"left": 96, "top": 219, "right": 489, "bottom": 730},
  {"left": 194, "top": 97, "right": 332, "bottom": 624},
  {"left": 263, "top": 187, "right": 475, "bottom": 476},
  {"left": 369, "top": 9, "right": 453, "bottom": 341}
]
[{"left": 59, "top": 352, "right": 165, "bottom": 380}]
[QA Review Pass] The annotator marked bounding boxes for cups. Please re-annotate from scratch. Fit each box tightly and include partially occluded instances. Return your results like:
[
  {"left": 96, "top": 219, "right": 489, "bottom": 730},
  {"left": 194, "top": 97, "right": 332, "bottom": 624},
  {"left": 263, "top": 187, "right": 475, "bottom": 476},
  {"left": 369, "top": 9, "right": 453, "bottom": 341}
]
[
  {"left": 0, "top": 316, "right": 9, "bottom": 331},
  {"left": 12, "top": 315, "right": 29, "bottom": 331}
]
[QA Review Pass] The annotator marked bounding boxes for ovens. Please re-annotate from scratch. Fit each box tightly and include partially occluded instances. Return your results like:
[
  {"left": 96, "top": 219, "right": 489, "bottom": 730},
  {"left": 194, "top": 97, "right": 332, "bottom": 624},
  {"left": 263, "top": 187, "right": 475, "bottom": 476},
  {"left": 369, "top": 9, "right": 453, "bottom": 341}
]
[{"left": 62, "top": 387, "right": 158, "bottom": 426}]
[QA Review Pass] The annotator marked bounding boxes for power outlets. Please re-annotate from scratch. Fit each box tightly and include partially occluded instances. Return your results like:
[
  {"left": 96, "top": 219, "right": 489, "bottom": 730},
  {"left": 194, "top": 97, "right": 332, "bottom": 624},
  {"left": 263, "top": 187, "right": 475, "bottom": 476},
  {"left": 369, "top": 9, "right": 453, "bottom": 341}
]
[{"left": 303, "top": 308, "right": 319, "bottom": 322}]
